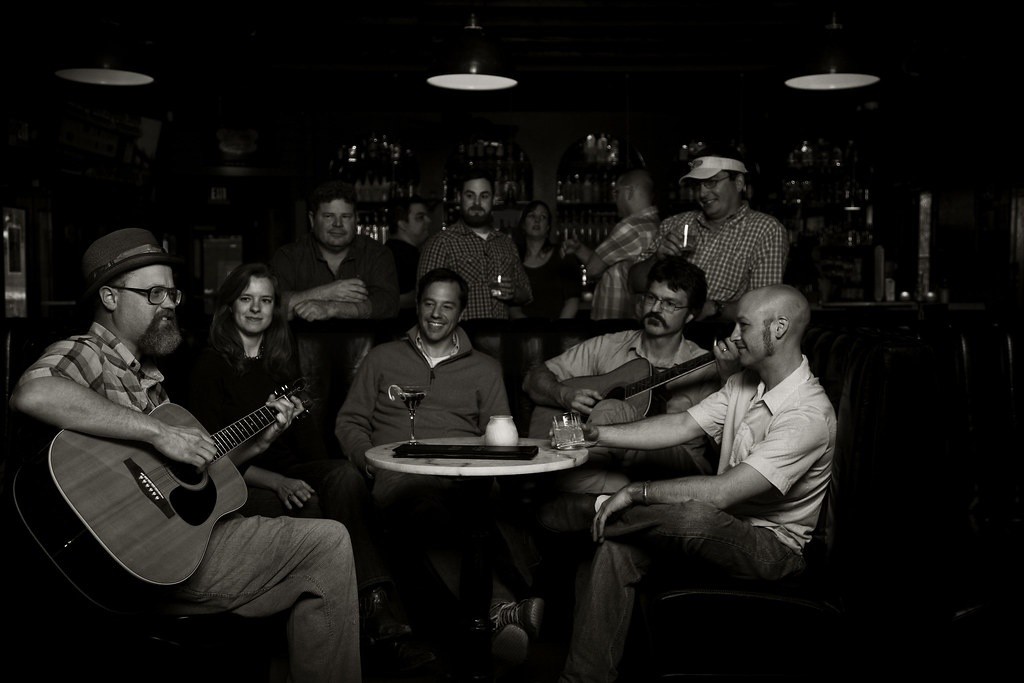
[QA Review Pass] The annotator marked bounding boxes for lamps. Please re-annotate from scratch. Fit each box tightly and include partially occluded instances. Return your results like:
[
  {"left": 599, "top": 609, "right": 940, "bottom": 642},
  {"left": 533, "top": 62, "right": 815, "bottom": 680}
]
[
  {"left": 54, "top": 0, "right": 156, "bottom": 86},
  {"left": 784, "top": 0, "right": 881, "bottom": 90},
  {"left": 426, "top": 0, "right": 519, "bottom": 91}
]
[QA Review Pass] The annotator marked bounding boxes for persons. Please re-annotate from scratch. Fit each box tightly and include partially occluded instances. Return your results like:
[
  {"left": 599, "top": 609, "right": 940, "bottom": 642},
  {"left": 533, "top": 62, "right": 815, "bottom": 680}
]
[
  {"left": 9, "top": 228, "right": 362, "bottom": 683},
  {"left": 191, "top": 145, "right": 789, "bottom": 683},
  {"left": 558, "top": 285, "right": 838, "bottom": 683}
]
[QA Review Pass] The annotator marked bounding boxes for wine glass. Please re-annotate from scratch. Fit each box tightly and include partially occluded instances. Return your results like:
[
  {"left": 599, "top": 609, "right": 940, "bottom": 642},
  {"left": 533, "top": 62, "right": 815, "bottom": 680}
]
[{"left": 391, "top": 385, "right": 430, "bottom": 445}]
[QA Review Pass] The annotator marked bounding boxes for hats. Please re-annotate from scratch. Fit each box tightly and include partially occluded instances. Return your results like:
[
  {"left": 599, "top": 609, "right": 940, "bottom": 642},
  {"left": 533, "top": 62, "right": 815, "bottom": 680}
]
[
  {"left": 679, "top": 156, "right": 748, "bottom": 187},
  {"left": 83, "top": 227, "right": 185, "bottom": 298}
]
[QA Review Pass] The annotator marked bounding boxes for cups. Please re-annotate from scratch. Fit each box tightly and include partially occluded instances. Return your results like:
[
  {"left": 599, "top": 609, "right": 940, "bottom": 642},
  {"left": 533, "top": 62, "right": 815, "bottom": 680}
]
[{"left": 551, "top": 411, "right": 586, "bottom": 450}]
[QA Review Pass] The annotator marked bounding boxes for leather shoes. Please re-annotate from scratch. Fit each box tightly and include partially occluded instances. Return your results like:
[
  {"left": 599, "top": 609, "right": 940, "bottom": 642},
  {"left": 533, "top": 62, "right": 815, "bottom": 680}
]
[{"left": 533, "top": 493, "right": 619, "bottom": 534}]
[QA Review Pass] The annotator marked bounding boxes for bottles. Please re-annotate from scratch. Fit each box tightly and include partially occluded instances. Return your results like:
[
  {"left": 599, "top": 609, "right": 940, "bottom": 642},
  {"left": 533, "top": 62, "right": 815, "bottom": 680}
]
[
  {"left": 484, "top": 414, "right": 518, "bottom": 446},
  {"left": 442, "top": 137, "right": 529, "bottom": 260},
  {"left": 162, "top": 233, "right": 176, "bottom": 256},
  {"left": 328, "top": 132, "right": 416, "bottom": 247},
  {"left": 554, "top": 132, "right": 620, "bottom": 261},
  {"left": 780, "top": 136, "right": 874, "bottom": 306},
  {"left": 665, "top": 136, "right": 753, "bottom": 222}
]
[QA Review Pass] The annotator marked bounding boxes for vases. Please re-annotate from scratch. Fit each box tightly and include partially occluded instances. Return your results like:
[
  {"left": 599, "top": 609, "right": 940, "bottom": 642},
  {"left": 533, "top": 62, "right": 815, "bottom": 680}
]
[{"left": 486, "top": 415, "right": 518, "bottom": 446}]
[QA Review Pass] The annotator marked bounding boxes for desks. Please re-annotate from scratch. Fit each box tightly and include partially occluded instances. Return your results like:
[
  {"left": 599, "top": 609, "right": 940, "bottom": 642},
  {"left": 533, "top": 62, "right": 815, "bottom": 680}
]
[{"left": 366, "top": 435, "right": 590, "bottom": 683}]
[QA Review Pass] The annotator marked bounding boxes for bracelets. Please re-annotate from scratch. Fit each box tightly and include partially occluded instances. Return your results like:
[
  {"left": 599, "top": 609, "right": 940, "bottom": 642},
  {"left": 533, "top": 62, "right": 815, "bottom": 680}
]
[{"left": 644, "top": 483, "right": 649, "bottom": 505}]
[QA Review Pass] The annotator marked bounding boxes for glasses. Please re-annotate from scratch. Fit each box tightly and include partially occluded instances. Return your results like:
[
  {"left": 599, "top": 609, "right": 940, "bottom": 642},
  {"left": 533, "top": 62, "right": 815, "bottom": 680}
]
[
  {"left": 611, "top": 185, "right": 631, "bottom": 201},
  {"left": 111, "top": 286, "right": 182, "bottom": 306},
  {"left": 640, "top": 293, "right": 689, "bottom": 313},
  {"left": 692, "top": 174, "right": 732, "bottom": 192}
]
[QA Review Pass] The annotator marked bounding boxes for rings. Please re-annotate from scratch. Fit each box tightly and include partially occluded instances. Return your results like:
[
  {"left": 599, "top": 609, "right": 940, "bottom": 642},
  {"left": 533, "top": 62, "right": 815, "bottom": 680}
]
[{"left": 722, "top": 348, "right": 727, "bottom": 352}]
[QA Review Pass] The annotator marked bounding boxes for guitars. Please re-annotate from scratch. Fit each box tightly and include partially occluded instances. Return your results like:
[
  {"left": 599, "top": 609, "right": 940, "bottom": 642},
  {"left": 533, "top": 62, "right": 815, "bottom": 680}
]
[
  {"left": 527, "top": 351, "right": 717, "bottom": 440},
  {"left": 9, "top": 372, "right": 317, "bottom": 618}
]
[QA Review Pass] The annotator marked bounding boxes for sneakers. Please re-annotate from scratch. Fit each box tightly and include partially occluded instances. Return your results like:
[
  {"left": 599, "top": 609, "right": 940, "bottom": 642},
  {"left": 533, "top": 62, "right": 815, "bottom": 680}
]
[
  {"left": 493, "top": 625, "right": 529, "bottom": 683},
  {"left": 491, "top": 597, "right": 544, "bottom": 632}
]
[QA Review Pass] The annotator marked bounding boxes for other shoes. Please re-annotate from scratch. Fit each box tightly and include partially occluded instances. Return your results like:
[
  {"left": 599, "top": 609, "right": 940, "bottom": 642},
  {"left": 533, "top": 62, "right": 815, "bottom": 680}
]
[
  {"left": 361, "top": 642, "right": 435, "bottom": 676},
  {"left": 361, "top": 581, "right": 411, "bottom": 644}
]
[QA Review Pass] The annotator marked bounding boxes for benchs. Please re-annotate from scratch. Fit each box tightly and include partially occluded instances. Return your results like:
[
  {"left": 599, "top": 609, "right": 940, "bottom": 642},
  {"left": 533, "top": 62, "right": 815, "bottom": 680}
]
[{"left": 30, "top": 313, "right": 970, "bottom": 683}]
